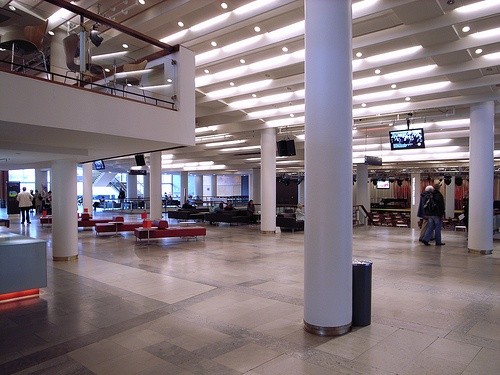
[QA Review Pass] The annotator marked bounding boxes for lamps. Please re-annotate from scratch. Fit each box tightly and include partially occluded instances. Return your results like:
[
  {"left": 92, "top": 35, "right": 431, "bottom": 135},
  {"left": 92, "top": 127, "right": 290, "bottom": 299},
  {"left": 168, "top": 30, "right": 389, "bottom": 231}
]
[
  {"left": 279, "top": 173, "right": 463, "bottom": 186},
  {"left": 90, "top": 22, "right": 103, "bottom": 47}
]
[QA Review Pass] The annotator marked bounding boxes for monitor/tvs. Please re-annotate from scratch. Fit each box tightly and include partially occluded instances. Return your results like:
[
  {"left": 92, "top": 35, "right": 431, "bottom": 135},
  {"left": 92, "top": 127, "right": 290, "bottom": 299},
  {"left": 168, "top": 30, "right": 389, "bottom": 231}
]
[
  {"left": 94, "top": 160, "right": 105, "bottom": 170},
  {"left": 377, "top": 180, "right": 390, "bottom": 189},
  {"left": 389, "top": 128, "right": 425, "bottom": 150}
]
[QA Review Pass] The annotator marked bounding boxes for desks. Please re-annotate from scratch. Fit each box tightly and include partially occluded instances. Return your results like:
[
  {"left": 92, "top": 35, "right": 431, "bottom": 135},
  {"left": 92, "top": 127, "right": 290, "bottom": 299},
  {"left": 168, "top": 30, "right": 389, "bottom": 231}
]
[
  {"left": 179, "top": 221, "right": 196, "bottom": 242},
  {"left": 108, "top": 222, "right": 123, "bottom": 238},
  {"left": 0, "top": 8, "right": 22, "bottom": 27}
]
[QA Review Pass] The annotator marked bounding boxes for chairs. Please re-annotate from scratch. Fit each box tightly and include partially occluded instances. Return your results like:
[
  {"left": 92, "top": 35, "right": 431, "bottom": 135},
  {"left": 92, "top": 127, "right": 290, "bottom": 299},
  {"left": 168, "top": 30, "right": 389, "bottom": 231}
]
[
  {"left": 116, "top": 59, "right": 147, "bottom": 103},
  {"left": 63, "top": 34, "right": 109, "bottom": 93},
  {"left": 0, "top": 18, "right": 49, "bottom": 80}
]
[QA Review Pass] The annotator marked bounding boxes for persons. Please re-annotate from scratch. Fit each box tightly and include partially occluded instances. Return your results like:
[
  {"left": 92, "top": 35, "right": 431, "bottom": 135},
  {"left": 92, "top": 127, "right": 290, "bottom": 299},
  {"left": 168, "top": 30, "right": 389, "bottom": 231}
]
[
  {"left": 30, "top": 188, "right": 52, "bottom": 215},
  {"left": 16, "top": 187, "right": 33, "bottom": 224},
  {"left": 417, "top": 180, "right": 445, "bottom": 246},
  {"left": 295, "top": 202, "right": 304, "bottom": 221},
  {"left": 194, "top": 196, "right": 203, "bottom": 206},
  {"left": 217, "top": 202, "right": 234, "bottom": 213},
  {"left": 247, "top": 200, "right": 255, "bottom": 215},
  {"left": 93, "top": 197, "right": 104, "bottom": 208},
  {"left": 182, "top": 201, "right": 191, "bottom": 209},
  {"left": 118, "top": 189, "right": 125, "bottom": 210}
]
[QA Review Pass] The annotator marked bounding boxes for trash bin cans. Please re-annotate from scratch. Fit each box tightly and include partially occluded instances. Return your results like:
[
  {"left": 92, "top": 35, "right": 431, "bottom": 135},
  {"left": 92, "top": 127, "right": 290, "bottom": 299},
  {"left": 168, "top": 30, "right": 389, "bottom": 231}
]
[{"left": 353, "top": 257, "right": 372, "bottom": 327}]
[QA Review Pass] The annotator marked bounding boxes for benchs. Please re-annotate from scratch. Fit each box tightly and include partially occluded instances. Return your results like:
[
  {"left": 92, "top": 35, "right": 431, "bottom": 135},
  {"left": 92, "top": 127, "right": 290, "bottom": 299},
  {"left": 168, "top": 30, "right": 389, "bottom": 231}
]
[
  {"left": 168, "top": 208, "right": 304, "bottom": 232},
  {"left": 95, "top": 221, "right": 155, "bottom": 237},
  {"left": 40, "top": 216, "right": 92, "bottom": 227},
  {"left": 78, "top": 219, "right": 116, "bottom": 227},
  {"left": 134, "top": 225, "right": 206, "bottom": 246}
]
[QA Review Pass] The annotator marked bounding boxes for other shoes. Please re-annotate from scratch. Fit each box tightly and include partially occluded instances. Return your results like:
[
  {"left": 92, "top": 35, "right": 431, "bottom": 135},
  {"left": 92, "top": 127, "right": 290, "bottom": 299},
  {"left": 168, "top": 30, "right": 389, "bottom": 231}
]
[
  {"left": 422, "top": 239, "right": 430, "bottom": 246},
  {"left": 418, "top": 239, "right": 422, "bottom": 241},
  {"left": 435, "top": 242, "right": 445, "bottom": 246},
  {"left": 430, "top": 238, "right": 435, "bottom": 241}
]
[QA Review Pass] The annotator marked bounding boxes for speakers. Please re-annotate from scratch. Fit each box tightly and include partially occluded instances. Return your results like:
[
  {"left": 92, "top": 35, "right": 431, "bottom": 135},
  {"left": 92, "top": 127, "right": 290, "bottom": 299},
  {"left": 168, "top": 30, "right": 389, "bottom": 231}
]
[
  {"left": 135, "top": 154, "right": 146, "bottom": 166},
  {"left": 277, "top": 140, "right": 296, "bottom": 156}
]
